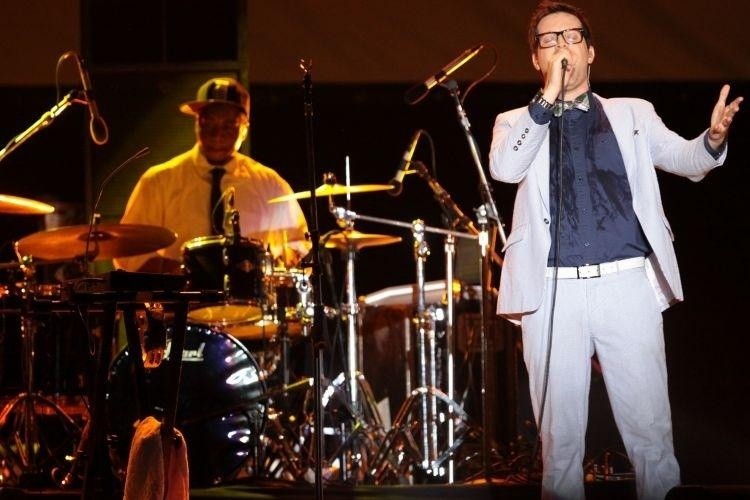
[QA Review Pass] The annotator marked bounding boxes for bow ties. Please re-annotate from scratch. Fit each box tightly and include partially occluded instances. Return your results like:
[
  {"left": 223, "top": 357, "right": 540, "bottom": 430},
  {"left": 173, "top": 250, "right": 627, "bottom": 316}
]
[{"left": 551, "top": 93, "right": 590, "bottom": 121}]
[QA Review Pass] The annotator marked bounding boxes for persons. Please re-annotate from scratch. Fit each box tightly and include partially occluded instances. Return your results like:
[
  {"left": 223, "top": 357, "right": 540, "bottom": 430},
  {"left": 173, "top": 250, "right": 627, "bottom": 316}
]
[
  {"left": 110, "top": 77, "right": 314, "bottom": 276},
  {"left": 487, "top": 1, "right": 744, "bottom": 500}
]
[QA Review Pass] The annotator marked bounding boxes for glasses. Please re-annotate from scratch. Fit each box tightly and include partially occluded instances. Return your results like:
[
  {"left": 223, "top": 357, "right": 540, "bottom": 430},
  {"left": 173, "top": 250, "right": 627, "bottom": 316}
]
[{"left": 534, "top": 28, "right": 586, "bottom": 49}]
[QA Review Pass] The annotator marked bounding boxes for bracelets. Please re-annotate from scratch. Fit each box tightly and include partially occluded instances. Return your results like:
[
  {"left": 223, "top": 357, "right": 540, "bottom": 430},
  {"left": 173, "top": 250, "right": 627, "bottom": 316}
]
[{"left": 531, "top": 87, "right": 553, "bottom": 109}]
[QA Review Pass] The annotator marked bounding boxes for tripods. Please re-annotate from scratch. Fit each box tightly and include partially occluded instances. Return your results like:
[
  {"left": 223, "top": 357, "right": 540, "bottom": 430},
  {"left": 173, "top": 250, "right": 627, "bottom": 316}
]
[
  {"left": 0, "top": 314, "right": 88, "bottom": 489},
  {"left": 271, "top": 207, "right": 543, "bottom": 486},
  {"left": 583, "top": 448, "right": 635, "bottom": 480}
]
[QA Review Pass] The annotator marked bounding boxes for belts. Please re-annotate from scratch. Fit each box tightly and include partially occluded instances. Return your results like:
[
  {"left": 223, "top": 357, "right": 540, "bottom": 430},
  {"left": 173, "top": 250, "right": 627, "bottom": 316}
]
[{"left": 547, "top": 253, "right": 652, "bottom": 280}]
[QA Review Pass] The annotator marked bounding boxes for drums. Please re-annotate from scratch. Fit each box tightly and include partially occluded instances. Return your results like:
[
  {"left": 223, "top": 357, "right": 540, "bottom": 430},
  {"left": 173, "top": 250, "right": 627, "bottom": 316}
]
[
  {"left": 105, "top": 321, "right": 269, "bottom": 485},
  {"left": 181, "top": 234, "right": 270, "bottom": 326},
  {"left": 1, "top": 262, "right": 90, "bottom": 312},
  {"left": 219, "top": 266, "right": 314, "bottom": 337}
]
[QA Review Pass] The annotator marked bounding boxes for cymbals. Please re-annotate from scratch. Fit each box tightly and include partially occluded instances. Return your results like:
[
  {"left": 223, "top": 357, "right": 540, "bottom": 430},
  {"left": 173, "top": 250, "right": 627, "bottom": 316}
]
[
  {"left": 19, "top": 223, "right": 179, "bottom": 260},
  {"left": 299, "top": 230, "right": 402, "bottom": 251},
  {"left": 266, "top": 184, "right": 400, "bottom": 204},
  {"left": 0, "top": 193, "right": 56, "bottom": 213}
]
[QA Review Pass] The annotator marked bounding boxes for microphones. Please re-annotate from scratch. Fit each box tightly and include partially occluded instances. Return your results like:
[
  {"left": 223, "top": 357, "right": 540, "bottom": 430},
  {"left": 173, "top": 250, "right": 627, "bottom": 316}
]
[
  {"left": 404, "top": 43, "right": 483, "bottom": 105},
  {"left": 62, "top": 147, "right": 187, "bottom": 293},
  {"left": 303, "top": 70, "right": 314, "bottom": 117},
  {"left": 387, "top": 131, "right": 421, "bottom": 197},
  {"left": 76, "top": 53, "right": 108, "bottom": 145}
]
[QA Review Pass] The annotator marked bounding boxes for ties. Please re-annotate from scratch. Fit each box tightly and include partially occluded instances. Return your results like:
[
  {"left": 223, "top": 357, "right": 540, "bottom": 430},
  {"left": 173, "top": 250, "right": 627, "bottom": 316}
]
[{"left": 207, "top": 166, "right": 228, "bottom": 241}]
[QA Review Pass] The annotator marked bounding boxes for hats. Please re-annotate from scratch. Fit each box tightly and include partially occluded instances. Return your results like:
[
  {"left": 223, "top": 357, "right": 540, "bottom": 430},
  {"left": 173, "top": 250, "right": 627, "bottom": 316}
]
[{"left": 178, "top": 75, "right": 253, "bottom": 120}]
